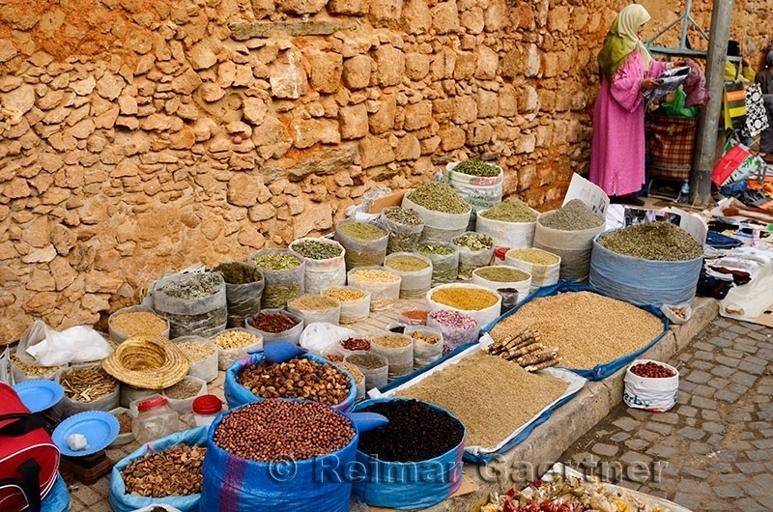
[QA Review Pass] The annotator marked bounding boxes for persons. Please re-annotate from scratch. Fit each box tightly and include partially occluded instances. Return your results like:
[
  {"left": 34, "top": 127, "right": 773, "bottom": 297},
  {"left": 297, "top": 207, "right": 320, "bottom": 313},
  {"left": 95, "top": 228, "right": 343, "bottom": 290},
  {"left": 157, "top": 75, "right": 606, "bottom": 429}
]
[{"left": 592, "top": 4, "right": 686, "bottom": 205}]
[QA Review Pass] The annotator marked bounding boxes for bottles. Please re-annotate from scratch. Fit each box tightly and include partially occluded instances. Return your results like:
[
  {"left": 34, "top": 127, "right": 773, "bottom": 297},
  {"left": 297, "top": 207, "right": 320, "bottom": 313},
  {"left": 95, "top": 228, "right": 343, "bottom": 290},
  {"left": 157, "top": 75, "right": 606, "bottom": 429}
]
[{"left": 131, "top": 394, "right": 225, "bottom": 445}]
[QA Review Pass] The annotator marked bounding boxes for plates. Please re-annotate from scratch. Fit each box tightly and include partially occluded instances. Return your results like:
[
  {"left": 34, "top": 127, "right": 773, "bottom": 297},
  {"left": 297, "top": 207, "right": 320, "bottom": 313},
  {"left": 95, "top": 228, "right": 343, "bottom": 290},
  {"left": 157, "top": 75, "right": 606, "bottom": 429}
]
[
  {"left": 10, "top": 379, "right": 64, "bottom": 415},
  {"left": 50, "top": 410, "right": 121, "bottom": 457}
]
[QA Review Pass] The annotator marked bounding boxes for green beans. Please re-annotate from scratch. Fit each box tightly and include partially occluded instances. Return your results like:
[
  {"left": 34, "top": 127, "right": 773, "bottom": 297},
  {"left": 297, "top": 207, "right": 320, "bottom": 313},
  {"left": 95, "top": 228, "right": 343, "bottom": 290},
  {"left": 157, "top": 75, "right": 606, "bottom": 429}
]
[{"left": 214, "top": 160, "right": 704, "bottom": 462}]
